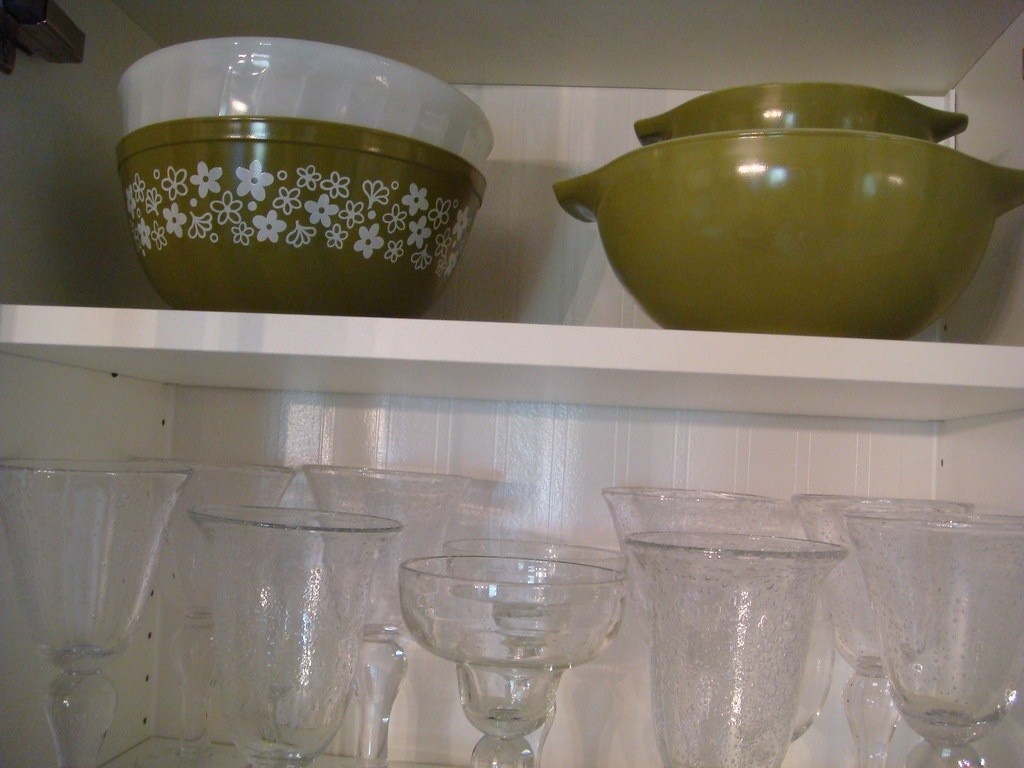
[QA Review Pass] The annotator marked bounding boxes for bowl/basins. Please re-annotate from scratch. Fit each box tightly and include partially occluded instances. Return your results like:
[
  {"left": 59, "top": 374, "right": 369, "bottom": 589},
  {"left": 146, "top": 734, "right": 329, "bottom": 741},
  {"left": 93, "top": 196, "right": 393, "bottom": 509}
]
[
  {"left": 117, "top": 116, "right": 487, "bottom": 317},
  {"left": 117, "top": 38, "right": 493, "bottom": 172},
  {"left": 555, "top": 127, "right": 1024, "bottom": 339},
  {"left": 634, "top": 82, "right": 967, "bottom": 146}
]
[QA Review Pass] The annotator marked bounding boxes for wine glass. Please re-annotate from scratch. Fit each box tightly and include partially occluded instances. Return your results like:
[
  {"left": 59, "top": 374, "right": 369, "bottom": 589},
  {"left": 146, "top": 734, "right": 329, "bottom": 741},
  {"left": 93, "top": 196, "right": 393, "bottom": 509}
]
[
  {"left": 305, "top": 465, "right": 473, "bottom": 768},
  {"left": 402, "top": 556, "right": 625, "bottom": 768},
  {"left": 0, "top": 458, "right": 191, "bottom": 768},
  {"left": 136, "top": 456, "right": 293, "bottom": 768},
  {"left": 792, "top": 492, "right": 973, "bottom": 768},
  {"left": 850, "top": 511, "right": 1024, "bottom": 768},
  {"left": 444, "top": 540, "right": 625, "bottom": 768}
]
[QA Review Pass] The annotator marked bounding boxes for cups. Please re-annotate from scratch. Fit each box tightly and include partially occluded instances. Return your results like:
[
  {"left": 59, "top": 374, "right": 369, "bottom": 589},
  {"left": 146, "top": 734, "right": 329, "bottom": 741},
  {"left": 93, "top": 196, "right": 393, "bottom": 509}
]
[
  {"left": 627, "top": 532, "right": 844, "bottom": 768},
  {"left": 602, "top": 485, "right": 775, "bottom": 651},
  {"left": 790, "top": 591, "right": 836, "bottom": 742},
  {"left": 185, "top": 503, "right": 400, "bottom": 768}
]
